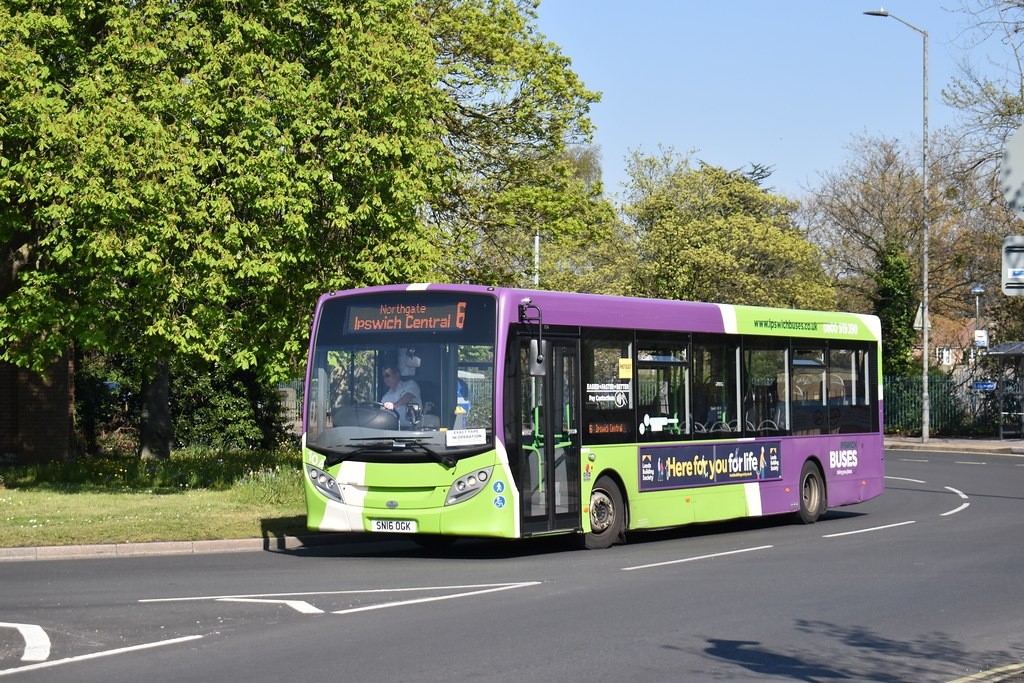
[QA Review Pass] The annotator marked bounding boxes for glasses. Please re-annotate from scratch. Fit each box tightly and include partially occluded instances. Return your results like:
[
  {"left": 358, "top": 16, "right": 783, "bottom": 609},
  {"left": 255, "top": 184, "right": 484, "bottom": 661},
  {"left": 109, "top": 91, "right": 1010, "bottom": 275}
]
[{"left": 382, "top": 373, "right": 390, "bottom": 379}]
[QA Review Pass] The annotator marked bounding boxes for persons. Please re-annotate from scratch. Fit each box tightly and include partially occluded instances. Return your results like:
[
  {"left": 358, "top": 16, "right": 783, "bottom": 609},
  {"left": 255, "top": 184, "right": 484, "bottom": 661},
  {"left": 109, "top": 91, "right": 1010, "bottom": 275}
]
[{"left": 379, "top": 363, "right": 424, "bottom": 430}]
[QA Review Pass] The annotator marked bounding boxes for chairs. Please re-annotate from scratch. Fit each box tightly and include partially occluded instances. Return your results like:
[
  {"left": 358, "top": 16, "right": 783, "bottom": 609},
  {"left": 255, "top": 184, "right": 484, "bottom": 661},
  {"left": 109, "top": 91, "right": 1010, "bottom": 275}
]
[
  {"left": 678, "top": 375, "right": 848, "bottom": 433},
  {"left": 419, "top": 381, "right": 436, "bottom": 415}
]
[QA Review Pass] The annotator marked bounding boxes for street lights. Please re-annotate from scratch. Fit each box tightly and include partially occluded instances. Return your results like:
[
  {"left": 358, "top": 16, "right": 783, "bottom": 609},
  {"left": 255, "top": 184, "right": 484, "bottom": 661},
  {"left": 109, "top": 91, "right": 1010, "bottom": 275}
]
[
  {"left": 861, "top": 8, "right": 930, "bottom": 444},
  {"left": 970, "top": 286, "right": 985, "bottom": 418}
]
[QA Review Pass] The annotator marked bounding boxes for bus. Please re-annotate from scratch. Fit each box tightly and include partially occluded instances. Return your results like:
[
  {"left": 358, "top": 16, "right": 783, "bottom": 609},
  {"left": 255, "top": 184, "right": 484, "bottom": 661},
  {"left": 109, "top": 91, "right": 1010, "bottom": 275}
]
[{"left": 298, "top": 282, "right": 888, "bottom": 553}]
